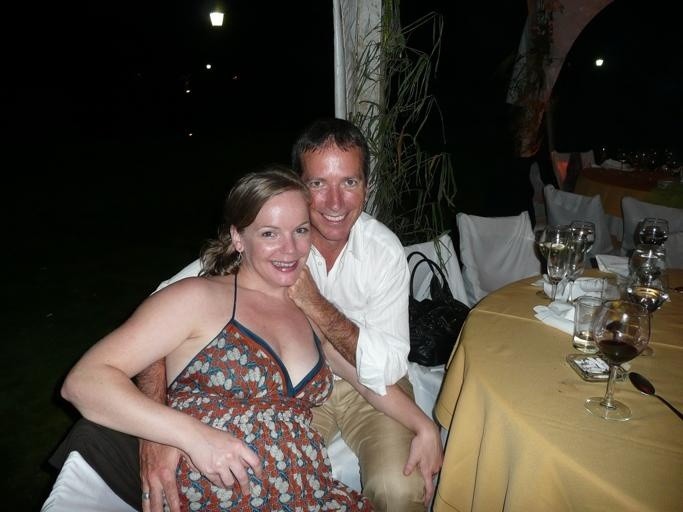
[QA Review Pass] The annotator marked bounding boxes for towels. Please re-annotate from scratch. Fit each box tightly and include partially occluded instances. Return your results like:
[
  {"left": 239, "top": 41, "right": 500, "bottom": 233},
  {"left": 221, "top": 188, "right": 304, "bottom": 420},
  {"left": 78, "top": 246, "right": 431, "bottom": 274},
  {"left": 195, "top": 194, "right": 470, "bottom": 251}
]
[
  {"left": 539, "top": 276, "right": 619, "bottom": 299},
  {"left": 531, "top": 297, "right": 579, "bottom": 336},
  {"left": 595, "top": 251, "right": 629, "bottom": 276}
]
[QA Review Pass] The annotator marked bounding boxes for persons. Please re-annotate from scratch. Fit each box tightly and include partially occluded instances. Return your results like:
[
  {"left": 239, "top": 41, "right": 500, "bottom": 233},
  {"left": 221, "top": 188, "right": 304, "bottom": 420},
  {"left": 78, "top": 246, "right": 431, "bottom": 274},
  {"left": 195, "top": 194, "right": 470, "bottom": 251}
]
[
  {"left": 60, "top": 169, "right": 444, "bottom": 512},
  {"left": 136, "top": 118, "right": 426, "bottom": 512}
]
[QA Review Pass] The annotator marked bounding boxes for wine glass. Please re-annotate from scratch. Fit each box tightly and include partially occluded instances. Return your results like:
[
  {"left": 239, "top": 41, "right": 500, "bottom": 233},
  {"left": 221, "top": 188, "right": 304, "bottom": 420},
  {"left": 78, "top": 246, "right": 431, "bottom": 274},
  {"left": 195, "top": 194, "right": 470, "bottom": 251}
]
[
  {"left": 584, "top": 299, "right": 650, "bottom": 424},
  {"left": 627, "top": 215, "right": 669, "bottom": 311},
  {"left": 537, "top": 219, "right": 596, "bottom": 305}
]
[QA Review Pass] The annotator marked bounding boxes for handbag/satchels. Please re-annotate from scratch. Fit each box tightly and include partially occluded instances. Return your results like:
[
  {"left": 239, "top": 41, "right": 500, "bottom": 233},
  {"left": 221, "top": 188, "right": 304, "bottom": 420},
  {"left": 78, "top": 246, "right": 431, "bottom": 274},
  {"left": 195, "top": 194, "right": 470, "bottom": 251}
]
[{"left": 407, "top": 251, "right": 470, "bottom": 367}]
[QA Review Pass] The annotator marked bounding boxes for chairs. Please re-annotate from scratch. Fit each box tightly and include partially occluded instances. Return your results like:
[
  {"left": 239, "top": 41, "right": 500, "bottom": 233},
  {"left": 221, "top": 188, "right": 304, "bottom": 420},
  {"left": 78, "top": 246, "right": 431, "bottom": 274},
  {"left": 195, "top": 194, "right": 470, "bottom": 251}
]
[
  {"left": 550, "top": 150, "right": 571, "bottom": 185},
  {"left": 619, "top": 195, "right": 683, "bottom": 269},
  {"left": 41, "top": 414, "right": 143, "bottom": 512},
  {"left": 331, "top": 433, "right": 361, "bottom": 491},
  {"left": 458, "top": 212, "right": 541, "bottom": 306},
  {"left": 396, "top": 232, "right": 470, "bottom": 454},
  {"left": 581, "top": 149, "right": 595, "bottom": 169},
  {"left": 543, "top": 182, "right": 614, "bottom": 265}
]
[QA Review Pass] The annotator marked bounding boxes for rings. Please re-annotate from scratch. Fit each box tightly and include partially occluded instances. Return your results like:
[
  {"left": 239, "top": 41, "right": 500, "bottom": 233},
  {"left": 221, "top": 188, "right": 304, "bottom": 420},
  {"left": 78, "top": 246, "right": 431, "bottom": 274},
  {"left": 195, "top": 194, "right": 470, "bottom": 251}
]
[{"left": 142, "top": 492, "right": 150, "bottom": 501}]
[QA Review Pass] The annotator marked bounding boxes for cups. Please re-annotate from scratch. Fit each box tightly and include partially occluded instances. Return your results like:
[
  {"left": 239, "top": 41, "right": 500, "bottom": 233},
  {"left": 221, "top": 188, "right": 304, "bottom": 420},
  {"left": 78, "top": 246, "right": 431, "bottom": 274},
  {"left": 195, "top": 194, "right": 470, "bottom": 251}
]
[
  {"left": 572, "top": 295, "right": 600, "bottom": 354},
  {"left": 603, "top": 268, "right": 625, "bottom": 297}
]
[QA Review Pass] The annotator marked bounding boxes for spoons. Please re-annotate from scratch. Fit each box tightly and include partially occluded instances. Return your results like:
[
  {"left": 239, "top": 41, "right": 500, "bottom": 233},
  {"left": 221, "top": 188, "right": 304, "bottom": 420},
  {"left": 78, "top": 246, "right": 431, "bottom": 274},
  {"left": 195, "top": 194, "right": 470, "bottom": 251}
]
[{"left": 629, "top": 371, "right": 682, "bottom": 422}]
